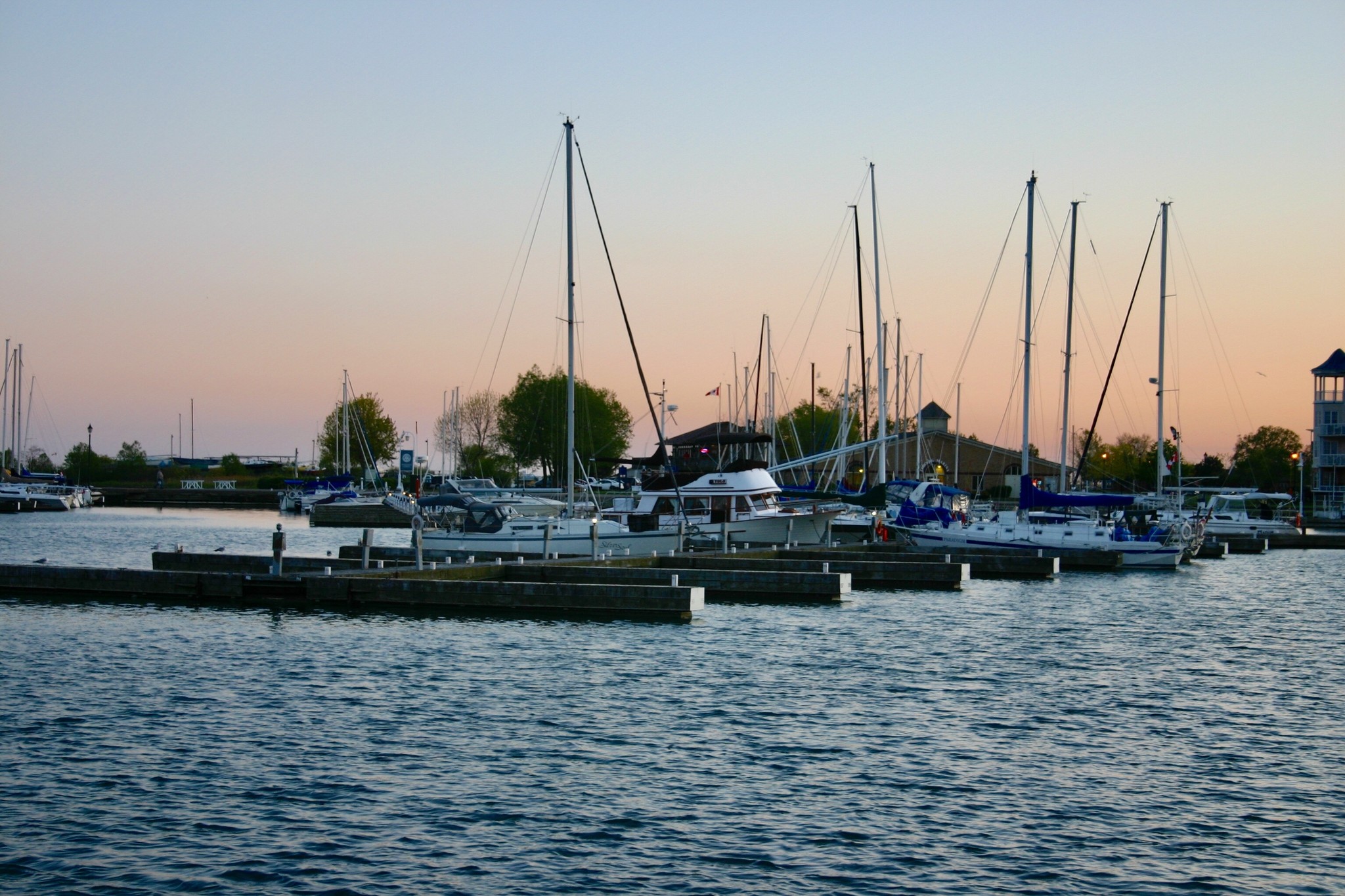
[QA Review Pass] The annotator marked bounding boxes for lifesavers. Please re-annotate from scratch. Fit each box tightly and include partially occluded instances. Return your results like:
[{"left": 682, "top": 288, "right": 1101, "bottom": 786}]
[
  {"left": 1180, "top": 524, "right": 1192, "bottom": 541},
  {"left": 1196, "top": 522, "right": 1204, "bottom": 536},
  {"left": 876, "top": 519, "right": 882, "bottom": 534},
  {"left": 951, "top": 511, "right": 966, "bottom": 523}
]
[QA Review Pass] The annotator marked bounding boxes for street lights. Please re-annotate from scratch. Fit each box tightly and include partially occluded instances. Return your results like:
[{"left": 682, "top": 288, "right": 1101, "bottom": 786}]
[
  {"left": 86, "top": 423, "right": 94, "bottom": 487},
  {"left": 404, "top": 431, "right": 415, "bottom": 453}
]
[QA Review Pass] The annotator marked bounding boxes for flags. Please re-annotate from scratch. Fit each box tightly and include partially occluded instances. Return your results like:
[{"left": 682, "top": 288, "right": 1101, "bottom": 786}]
[
  {"left": 705, "top": 387, "right": 719, "bottom": 396},
  {"left": 1166, "top": 452, "right": 1177, "bottom": 470}
]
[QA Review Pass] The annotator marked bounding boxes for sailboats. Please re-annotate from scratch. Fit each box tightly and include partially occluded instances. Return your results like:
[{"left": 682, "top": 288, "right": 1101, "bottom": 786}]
[
  {"left": 278, "top": 116, "right": 1304, "bottom": 571},
  {"left": 0, "top": 338, "right": 105, "bottom": 511}
]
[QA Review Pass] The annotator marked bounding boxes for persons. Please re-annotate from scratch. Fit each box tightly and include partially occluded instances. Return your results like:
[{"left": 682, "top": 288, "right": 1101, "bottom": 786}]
[
  {"left": 683, "top": 452, "right": 690, "bottom": 462},
  {"left": 153, "top": 468, "right": 164, "bottom": 489}
]
